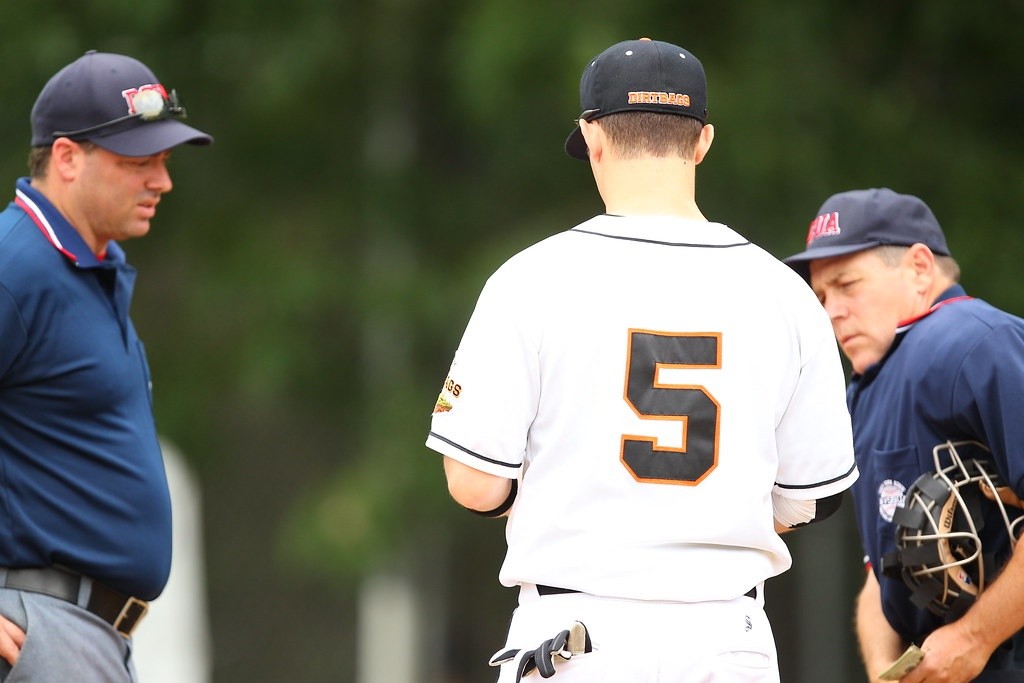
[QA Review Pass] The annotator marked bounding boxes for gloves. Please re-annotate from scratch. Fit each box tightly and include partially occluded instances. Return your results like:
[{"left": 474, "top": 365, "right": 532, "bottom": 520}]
[{"left": 489, "top": 621, "right": 592, "bottom": 683}]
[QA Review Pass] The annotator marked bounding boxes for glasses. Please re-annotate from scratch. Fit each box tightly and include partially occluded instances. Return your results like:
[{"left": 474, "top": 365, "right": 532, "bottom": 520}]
[{"left": 52, "top": 89, "right": 187, "bottom": 138}]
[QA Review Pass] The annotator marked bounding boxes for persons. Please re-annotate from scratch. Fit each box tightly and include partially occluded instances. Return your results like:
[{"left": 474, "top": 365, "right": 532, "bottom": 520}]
[
  {"left": 426, "top": 35, "right": 859, "bottom": 683},
  {"left": 0, "top": 49, "right": 211, "bottom": 683},
  {"left": 779, "top": 187, "right": 1023, "bottom": 682}
]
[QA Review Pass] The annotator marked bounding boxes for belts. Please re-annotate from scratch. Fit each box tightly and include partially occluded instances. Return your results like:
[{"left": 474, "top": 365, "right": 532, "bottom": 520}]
[
  {"left": 535, "top": 585, "right": 758, "bottom": 603},
  {"left": 5, "top": 565, "right": 148, "bottom": 639}
]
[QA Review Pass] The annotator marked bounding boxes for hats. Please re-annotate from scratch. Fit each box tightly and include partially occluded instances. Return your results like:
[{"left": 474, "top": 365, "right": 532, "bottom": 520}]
[
  {"left": 565, "top": 38, "right": 707, "bottom": 161},
  {"left": 782, "top": 187, "right": 951, "bottom": 285},
  {"left": 30, "top": 50, "right": 213, "bottom": 156}
]
